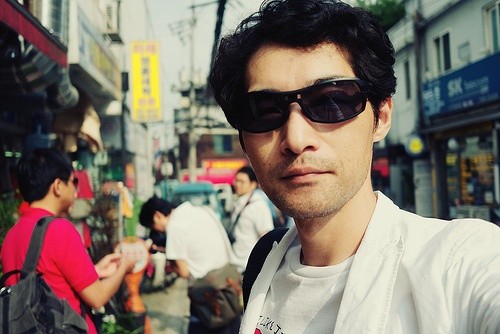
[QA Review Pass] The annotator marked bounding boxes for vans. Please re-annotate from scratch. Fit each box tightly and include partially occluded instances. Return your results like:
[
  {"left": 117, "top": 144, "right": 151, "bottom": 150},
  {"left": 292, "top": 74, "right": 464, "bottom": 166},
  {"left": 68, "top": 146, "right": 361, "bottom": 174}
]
[{"left": 158, "top": 178, "right": 227, "bottom": 226}]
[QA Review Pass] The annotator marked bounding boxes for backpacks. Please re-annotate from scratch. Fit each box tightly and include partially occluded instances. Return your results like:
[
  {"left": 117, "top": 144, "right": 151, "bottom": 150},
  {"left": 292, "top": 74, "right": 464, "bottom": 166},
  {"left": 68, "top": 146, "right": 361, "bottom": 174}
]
[{"left": 0, "top": 216, "right": 89, "bottom": 334}]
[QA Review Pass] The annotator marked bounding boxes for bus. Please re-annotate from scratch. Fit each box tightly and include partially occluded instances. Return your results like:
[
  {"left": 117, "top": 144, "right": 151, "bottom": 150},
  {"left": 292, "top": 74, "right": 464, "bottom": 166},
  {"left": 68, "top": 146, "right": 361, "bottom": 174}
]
[{"left": 179, "top": 125, "right": 252, "bottom": 192}]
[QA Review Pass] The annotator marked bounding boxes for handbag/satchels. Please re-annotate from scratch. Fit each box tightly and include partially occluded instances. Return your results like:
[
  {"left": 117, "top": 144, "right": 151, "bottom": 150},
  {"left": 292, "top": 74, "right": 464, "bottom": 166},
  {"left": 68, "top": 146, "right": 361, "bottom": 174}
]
[{"left": 188, "top": 263, "right": 244, "bottom": 334}]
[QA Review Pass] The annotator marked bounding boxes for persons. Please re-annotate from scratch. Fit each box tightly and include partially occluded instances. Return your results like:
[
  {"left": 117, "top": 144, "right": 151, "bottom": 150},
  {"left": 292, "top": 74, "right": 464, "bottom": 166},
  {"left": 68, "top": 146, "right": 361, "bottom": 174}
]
[
  {"left": 207, "top": 0, "right": 500, "bottom": 334},
  {"left": 138, "top": 196, "right": 253, "bottom": 334},
  {"left": 0, "top": 145, "right": 135, "bottom": 334},
  {"left": 227, "top": 166, "right": 275, "bottom": 270}
]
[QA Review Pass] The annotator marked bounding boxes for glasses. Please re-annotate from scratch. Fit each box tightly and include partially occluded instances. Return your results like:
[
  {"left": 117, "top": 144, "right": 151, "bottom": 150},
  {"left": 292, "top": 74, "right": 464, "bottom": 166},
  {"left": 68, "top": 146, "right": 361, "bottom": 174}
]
[
  {"left": 69, "top": 178, "right": 78, "bottom": 185},
  {"left": 230, "top": 78, "right": 367, "bottom": 134}
]
